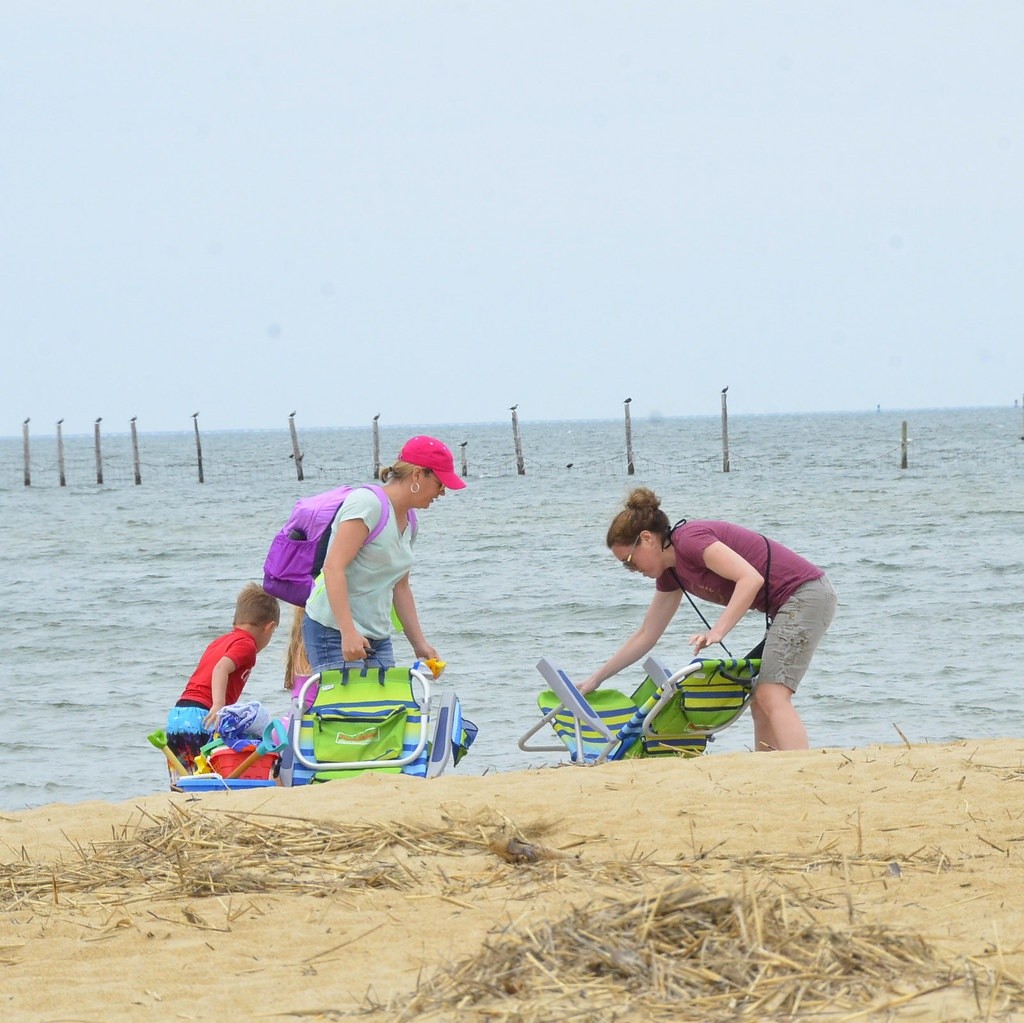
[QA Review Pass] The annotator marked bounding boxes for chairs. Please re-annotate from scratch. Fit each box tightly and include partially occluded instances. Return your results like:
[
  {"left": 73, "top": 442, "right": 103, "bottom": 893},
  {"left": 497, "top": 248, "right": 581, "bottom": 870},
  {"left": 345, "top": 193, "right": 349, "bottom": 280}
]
[
  {"left": 518, "top": 657, "right": 760, "bottom": 761},
  {"left": 289, "top": 667, "right": 479, "bottom": 787}
]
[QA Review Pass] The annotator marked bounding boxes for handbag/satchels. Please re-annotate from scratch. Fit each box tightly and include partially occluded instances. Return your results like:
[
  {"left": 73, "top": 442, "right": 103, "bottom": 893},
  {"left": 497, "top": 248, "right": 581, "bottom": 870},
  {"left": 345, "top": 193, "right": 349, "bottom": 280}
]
[{"left": 742, "top": 637, "right": 766, "bottom": 660}]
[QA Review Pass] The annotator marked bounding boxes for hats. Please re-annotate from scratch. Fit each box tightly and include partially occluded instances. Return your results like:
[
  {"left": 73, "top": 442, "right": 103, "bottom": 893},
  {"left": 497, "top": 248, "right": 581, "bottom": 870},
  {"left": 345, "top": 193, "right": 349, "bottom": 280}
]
[{"left": 398, "top": 436, "right": 467, "bottom": 490}]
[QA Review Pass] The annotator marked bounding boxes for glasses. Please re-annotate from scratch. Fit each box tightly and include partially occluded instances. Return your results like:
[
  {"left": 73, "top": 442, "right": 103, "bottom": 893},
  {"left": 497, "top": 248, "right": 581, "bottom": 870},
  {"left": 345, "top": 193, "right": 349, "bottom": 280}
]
[
  {"left": 623, "top": 535, "right": 640, "bottom": 572},
  {"left": 426, "top": 471, "right": 446, "bottom": 492}
]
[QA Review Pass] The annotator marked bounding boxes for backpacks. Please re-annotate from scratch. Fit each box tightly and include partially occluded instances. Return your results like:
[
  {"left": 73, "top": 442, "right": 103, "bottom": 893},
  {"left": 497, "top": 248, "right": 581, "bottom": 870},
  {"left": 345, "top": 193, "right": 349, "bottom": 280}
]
[{"left": 263, "top": 485, "right": 416, "bottom": 608}]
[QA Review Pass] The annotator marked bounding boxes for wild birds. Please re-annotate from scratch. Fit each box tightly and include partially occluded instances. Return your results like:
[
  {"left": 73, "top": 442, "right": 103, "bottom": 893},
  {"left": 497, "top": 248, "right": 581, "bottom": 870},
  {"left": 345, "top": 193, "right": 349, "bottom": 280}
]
[
  {"left": 508, "top": 404, "right": 519, "bottom": 412},
  {"left": 289, "top": 411, "right": 296, "bottom": 417},
  {"left": 129, "top": 414, "right": 137, "bottom": 422},
  {"left": 23, "top": 416, "right": 31, "bottom": 424},
  {"left": 95, "top": 416, "right": 102, "bottom": 424},
  {"left": 624, "top": 397, "right": 631, "bottom": 403},
  {"left": 458, "top": 441, "right": 467, "bottom": 447},
  {"left": 721, "top": 386, "right": 728, "bottom": 394},
  {"left": 373, "top": 413, "right": 381, "bottom": 421},
  {"left": 190, "top": 411, "right": 199, "bottom": 417},
  {"left": 57, "top": 418, "right": 64, "bottom": 424}
]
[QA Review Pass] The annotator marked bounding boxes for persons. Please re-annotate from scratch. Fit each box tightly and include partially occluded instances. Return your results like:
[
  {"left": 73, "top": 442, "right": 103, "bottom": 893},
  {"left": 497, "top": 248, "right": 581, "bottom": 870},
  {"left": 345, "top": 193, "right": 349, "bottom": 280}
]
[
  {"left": 300, "top": 434, "right": 466, "bottom": 675},
  {"left": 572, "top": 487, "right": 838, "bottom": 748},
  {"left": 166, "top": 582, "right": 280, "bottom": 791}
]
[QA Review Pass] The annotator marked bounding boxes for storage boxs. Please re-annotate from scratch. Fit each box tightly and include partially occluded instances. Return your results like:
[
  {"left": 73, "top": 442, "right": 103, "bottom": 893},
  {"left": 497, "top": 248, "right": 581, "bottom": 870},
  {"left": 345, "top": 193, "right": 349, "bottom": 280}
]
[
  {"left": 174, "top": 780, "right": 277, "bottom": 791},
  {"left": 206, "top": 748, "right": 278, "bottom": 780}
]
